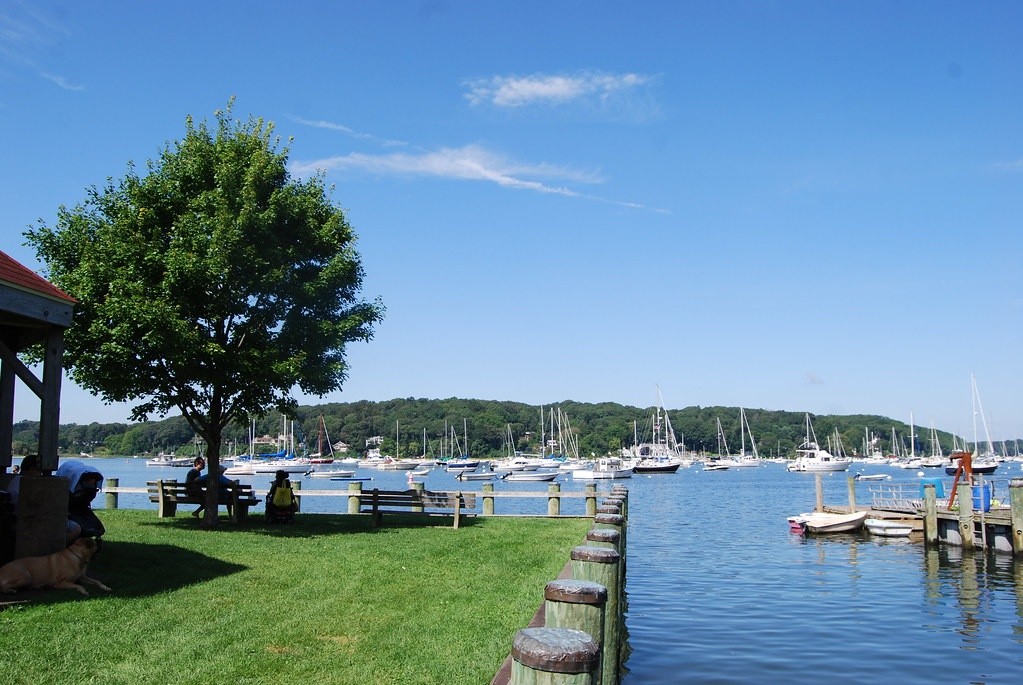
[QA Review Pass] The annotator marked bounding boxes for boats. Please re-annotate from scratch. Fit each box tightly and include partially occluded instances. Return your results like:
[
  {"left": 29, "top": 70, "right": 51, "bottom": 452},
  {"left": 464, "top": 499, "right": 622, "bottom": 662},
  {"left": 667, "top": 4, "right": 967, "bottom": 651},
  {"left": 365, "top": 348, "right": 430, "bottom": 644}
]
[
  {"left": 309, "top": 470, "right": 357, "bottom": 478},
  {"left": 788, "top": 511, "right": 866, "bottom": 535},
  {"left": 147, "top": 454, "right": 174, "bottom": 467},
  {"left": 224, "top": 440, "right": 247, "bottom": 468},
  {"left": 330, "top": 477, "right": 374, "bottom": 482},
  {"left": 235, "top": 458, "right": 313, "bottom": 473},
  {"left": 854, "top": 472, "right": 888, "bottom": 481},
  {"left": 170, "top": 458, "right": 198, "bottom": 467},
  {"left": 864, "top": 519, "right": 910, "bottom": 538},
  {"left": 80, "top": 452, "right": 92, "bottom": 458},
  {"left": 225, "top": 466, "right": 257, "bottom": 475}
]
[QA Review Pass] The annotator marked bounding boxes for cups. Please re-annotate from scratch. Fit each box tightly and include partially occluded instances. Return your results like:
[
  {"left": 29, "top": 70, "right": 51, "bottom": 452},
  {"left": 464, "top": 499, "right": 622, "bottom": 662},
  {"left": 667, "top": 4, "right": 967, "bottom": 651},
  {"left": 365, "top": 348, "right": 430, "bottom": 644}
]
[{"left": 251, "top": 491, "right": 255, "bottom": 497}]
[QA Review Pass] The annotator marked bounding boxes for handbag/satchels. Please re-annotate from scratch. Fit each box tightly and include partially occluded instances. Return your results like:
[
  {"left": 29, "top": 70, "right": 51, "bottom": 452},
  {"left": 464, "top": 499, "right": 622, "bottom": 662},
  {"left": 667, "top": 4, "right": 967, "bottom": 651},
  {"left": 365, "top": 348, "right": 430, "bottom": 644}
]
[{"left": 273, "top": 481, "right": 291, "bottom": 507}]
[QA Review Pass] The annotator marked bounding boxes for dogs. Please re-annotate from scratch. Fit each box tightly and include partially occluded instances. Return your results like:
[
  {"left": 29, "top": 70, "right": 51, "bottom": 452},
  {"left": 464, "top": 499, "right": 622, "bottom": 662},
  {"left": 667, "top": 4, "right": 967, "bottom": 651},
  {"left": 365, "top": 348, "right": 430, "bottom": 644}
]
[{"left": 0, "top": 536, "right": 112, "bottom": 605}]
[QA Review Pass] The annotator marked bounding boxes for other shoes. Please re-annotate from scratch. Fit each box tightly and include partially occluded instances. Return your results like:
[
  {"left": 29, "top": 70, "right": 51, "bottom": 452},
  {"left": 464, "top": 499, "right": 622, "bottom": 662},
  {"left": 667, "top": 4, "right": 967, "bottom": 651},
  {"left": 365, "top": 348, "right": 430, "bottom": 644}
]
[
  {"left": 192, "top": 511, "right": 200, "bottom": 520},
  {"left": 229, "top": 515, "right": 232, "bottom": 520}
]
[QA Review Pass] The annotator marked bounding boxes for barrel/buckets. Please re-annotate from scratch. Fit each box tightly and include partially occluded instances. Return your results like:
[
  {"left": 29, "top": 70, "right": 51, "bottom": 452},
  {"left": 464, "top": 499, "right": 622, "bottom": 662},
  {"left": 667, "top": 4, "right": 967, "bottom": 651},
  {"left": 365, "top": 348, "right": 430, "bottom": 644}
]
[{"left": 973, "top": 485, "right": 990, "bottom": 513}]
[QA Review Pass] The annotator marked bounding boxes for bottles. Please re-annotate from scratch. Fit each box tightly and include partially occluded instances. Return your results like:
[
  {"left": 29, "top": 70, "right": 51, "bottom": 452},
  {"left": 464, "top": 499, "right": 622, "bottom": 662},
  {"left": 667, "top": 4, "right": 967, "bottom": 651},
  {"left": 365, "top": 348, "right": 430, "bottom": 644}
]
[
  {"left": 235, "top": 480, "right": 239, "bottom": 491},
  {"left": 268, "top": 493, "right": 270, "bottom": 502},
  {"left": 409, "top": 473, "right": 413, "bottom": 487}
]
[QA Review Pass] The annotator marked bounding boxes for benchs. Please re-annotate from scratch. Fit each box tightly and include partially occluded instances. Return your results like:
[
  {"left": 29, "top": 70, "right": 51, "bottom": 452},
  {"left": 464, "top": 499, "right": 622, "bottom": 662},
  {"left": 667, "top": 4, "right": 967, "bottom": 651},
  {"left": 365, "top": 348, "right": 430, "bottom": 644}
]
[
  {"left": 0, "top": 490, "right": 17, "bottom": 564},
  {"left": 147, "top": 479, "right": 261, "bottom": 523},
  {"left": 360, "top": 488, "right": 477, "bottom": 529}
]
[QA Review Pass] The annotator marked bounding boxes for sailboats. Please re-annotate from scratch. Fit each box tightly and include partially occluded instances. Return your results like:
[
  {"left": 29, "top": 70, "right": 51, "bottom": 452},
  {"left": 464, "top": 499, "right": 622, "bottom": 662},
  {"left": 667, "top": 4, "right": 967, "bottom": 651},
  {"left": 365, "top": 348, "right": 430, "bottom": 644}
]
[
  {"left": 785, "top": 373, "right": 1023, "bottom": 475},
  {"left": 240, "top": 413, "right": 335, "bottom": 466},
  {"left": 442, "top": 384, "right": 786, "bottom": 482},
  {"left": 358, "top": 410, "right": 443, "bottom": 481}
]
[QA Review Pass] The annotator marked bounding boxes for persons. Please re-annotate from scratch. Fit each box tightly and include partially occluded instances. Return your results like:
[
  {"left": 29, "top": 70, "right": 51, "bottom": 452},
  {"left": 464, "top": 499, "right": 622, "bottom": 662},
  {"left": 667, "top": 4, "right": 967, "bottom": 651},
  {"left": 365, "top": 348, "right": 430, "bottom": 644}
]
[
  {"left": 267, "top": 470, "right": 294, "bottom": 524},
  {"left": 194, "top": 466, "right": 241, "bottom": 520},
  {"left": 7, "top": 455, "right": 80, "bottom": 547},
  {"left": 186, "top": 457, "right": 206, "bottom": 519}
]
